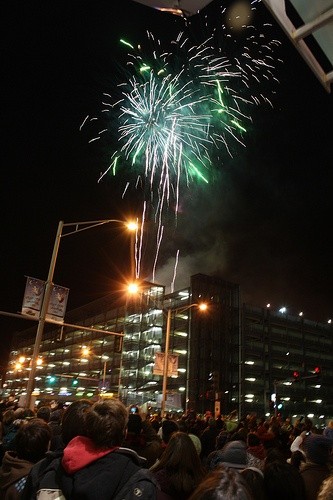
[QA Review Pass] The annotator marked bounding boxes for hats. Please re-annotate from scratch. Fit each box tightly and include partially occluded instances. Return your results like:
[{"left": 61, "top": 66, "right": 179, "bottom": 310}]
[
  {"left": 246, "top": 432, "right": 269, "bottom": 462},
  {"left": 163, "top": 420, "right": 177, "bottom": 435},
  {"left": 253, "top": 426, "right": 278, "bottom": 449},
  {"left": 223, "top": 440, "right": 249, "bottom": 464},
  {"left": 303, "top": 433, "right": 333, "bottom": 464}
]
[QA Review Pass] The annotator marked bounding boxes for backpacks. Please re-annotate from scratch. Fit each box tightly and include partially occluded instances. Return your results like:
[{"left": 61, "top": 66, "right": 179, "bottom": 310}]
[{"left": 209, "top": 469, "right": 254, "bottom": 500}]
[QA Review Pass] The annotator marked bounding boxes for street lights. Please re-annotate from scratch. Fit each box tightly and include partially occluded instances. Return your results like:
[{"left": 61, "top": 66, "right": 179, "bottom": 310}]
[
  {"left": 23, "top": 218, "right": 141, "bottom": 409},
  {"left": 160, "top": 302, "right": 210, "bottom": 418}
]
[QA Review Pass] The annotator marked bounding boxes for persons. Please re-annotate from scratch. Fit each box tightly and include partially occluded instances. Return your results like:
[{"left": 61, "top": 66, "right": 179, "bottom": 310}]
[
  {"left": 0, "top": 396, "right": 146, "bottom": 500},
  {"left": 114, "top": 411, "right": 256, "bottom": 500},
  {"left": 239, "top": 412, "right": 333, "bottom": 500}
]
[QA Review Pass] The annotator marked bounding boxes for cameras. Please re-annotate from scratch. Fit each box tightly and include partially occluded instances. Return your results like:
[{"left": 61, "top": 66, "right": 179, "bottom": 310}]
[
  {"left": 305, "top": 432, "right": 310, "bottom": 435},
  {"left": 130, "top": 407, "right": 138, "bottom": 413}
]
